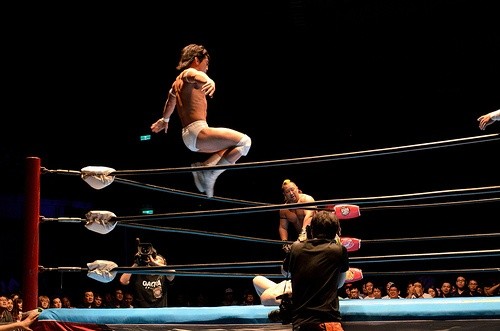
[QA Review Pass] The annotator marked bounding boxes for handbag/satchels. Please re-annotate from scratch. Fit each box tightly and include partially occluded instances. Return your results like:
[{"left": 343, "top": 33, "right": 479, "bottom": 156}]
[{"left": 319, "top": 322, "right": 344, "bottom": 331}]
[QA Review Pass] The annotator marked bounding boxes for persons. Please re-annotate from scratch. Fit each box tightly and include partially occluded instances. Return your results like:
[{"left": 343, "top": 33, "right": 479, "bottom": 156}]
[
  {"left": 0, "top": 180, "right": 500, "bottom": 331},
  {"left": 150, "top": 44, "right": 251, "bottom": 197},
  {"left": 477, "top": 109, "right": 500, "bottom": 130}
]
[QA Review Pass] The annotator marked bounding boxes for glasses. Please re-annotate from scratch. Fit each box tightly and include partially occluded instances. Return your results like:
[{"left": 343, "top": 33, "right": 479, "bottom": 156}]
[{"left": 456, "top": 279, "right": 465, "bottom": 282}]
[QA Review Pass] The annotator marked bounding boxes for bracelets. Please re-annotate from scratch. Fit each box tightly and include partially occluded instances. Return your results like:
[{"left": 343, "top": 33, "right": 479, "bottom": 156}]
[{"left": 161, "top": 117, "right": 169, "bottom": 122}]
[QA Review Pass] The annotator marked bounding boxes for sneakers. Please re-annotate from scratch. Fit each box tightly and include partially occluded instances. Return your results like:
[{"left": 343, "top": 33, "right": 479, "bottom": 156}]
[
  {"left": 191, "top": 162, "right": 205, "bottom": 192},
  {"left": 197, "top": 170, "right": 215, "bottom": 198}
]
